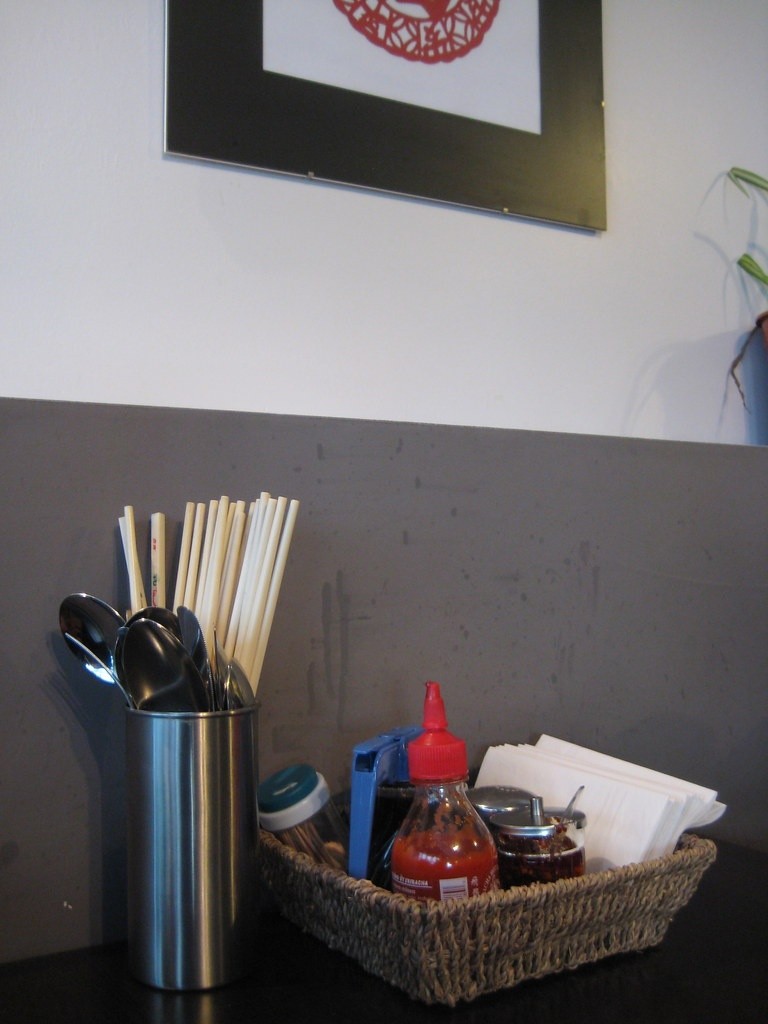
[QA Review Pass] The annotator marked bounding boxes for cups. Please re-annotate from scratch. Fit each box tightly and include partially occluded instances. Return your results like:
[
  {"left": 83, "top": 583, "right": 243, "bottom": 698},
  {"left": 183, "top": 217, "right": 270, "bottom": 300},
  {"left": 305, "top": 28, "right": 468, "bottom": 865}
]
[{"left": 122, "top": 703, "right": 262, "bottom": 992}]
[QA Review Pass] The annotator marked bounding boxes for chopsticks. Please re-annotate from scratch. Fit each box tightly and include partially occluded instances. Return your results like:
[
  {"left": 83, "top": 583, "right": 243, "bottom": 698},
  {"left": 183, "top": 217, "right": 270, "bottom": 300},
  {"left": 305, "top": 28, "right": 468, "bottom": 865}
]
[{"left": 117, "top": 488, "right": 300, "bottom": 701}]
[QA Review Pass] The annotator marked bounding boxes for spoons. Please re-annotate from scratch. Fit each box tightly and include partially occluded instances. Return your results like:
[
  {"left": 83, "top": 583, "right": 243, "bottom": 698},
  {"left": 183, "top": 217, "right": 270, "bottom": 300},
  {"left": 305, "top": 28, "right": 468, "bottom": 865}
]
[{"left": 57, "top": 593, "right": 257, "bottom": 708}]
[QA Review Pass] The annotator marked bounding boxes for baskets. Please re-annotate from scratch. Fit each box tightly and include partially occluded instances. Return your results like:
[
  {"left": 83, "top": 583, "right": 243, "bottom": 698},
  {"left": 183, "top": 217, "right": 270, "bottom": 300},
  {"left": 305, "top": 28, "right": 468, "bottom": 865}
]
[{"left": 257, "top": 777, "right": 717, "bottom": 1004}]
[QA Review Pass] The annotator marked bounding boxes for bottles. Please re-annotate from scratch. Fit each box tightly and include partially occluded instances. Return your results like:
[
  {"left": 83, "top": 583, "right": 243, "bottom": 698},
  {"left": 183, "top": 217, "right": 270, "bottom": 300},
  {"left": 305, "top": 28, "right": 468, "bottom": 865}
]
[
  {"left": 463, "top": 784, "right": 588, "bottom": 892},
  {"left": 387, "top": 680, "right": 504, "bottom": 904}
]
